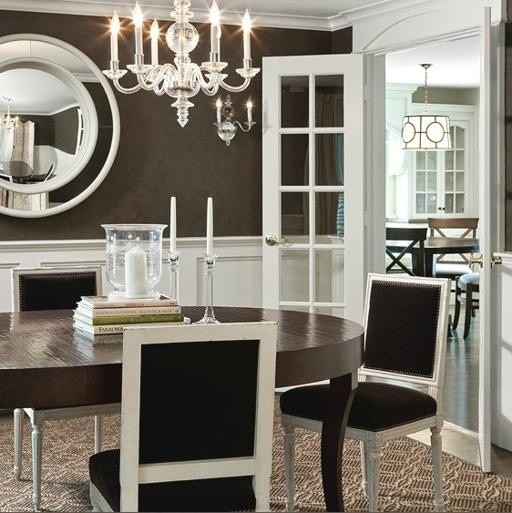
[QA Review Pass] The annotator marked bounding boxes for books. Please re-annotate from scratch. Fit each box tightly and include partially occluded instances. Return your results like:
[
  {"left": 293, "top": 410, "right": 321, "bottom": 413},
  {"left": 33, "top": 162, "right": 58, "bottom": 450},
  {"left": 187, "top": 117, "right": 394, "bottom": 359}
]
[
  {"left": 72, "top": 295, "right": 192, "bottom": 334},
  {"left": 73, "top": 328, "right": 123, "bottom": 360}
]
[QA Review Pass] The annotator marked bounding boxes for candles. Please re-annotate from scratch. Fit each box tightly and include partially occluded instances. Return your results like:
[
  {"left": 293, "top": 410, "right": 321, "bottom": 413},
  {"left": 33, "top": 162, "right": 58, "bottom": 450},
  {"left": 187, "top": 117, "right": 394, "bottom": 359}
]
[
  {"left": 169, "top": 197, "right": 177, "bottom": 258},
  {"left": 206, "top": 196, "right": 214, "bottom": 258}
]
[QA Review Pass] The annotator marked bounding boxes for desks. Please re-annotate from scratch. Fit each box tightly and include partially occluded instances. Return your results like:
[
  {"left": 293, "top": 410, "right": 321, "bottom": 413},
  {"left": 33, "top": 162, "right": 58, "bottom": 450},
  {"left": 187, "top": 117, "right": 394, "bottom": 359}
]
[{"left": 0, "top": 302, "right": 362, "bottom": 512}]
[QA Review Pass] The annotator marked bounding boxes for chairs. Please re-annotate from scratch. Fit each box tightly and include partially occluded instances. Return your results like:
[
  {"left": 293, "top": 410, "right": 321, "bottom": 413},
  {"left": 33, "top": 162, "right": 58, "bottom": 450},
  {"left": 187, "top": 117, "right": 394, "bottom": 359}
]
[
  {"left": 385, "top": 217, "right": 483, "bottom": 341},
  {"left": 280, "top": 273, "right": 450, "bottom": 513},
  {"left": 11, "top": 264, "right": 120, "bottom": 512},
  {"left": 87, "top": 321, "right": 278, "bottom": 512}
]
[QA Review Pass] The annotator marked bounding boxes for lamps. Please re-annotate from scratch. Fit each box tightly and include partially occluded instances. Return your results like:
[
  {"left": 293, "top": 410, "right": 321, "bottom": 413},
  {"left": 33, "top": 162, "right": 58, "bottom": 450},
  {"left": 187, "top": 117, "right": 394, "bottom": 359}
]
[
  {"left": 102, "top": 0, "right": 262, "bottom": 129},
  {"left": 0, "top": 97, "right": 35, "bottom": 180},
  {"left": 400, "top": 65, "right": 455, "bottom": 150}
]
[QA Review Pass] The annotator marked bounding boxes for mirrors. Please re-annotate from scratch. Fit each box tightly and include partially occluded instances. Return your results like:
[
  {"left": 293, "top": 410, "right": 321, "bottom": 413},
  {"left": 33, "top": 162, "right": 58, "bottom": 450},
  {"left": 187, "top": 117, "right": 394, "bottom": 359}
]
[{"left": 0, "top": 33, "right": 121, "bottom": 219}]
